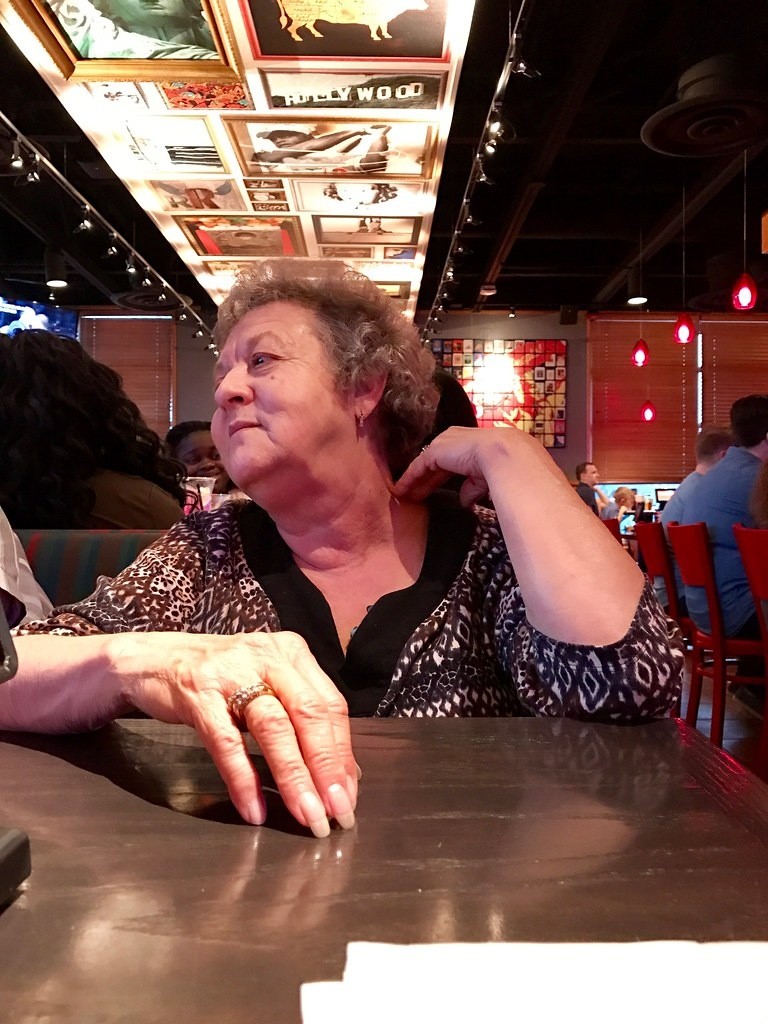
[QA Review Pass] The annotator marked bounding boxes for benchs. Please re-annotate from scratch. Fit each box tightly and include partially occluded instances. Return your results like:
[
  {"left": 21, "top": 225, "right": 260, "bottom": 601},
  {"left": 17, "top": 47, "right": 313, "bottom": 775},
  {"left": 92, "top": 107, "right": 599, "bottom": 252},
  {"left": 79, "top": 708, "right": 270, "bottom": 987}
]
[{"left": 13, "top": 527, "right": 170, "bottom": 615}]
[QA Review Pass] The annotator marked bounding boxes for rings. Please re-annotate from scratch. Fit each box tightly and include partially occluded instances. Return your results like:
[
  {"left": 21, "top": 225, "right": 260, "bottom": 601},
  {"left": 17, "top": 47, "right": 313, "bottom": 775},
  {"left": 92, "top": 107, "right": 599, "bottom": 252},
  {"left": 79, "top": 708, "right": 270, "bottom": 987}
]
[
  {"left": 420, "top": 444, "right": 430, "bottom": 453},
  {"left": 227, "top": 681, "right": 277, "bottom": 725}
]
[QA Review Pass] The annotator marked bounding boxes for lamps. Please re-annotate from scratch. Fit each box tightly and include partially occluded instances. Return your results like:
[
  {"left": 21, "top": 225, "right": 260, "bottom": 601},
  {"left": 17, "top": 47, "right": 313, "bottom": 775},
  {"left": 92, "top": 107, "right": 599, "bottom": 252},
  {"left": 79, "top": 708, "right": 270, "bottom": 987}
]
[
  {"left": 733, "top": 145, "right": 757, "bottom": 309},
  {"left": 421, "top": 16, "right": 535, "bottom": 359},
  {"left": 558, "top": 304, "right": 579, "bottom": 324},
  {"left": 673, "top": 179, "right": 695, "bottom": 343},
  {"left": 9, "top": 139, "right": 222, "bottom": 356},
  {"left": 639, "top": 255, "right": 658, "bottom": 423},
  {"left": 630, "top": 231, "right": 652, "bottom": 368},
  {"left": 627, "top": 267, "right": 649, "bottom": 306}
]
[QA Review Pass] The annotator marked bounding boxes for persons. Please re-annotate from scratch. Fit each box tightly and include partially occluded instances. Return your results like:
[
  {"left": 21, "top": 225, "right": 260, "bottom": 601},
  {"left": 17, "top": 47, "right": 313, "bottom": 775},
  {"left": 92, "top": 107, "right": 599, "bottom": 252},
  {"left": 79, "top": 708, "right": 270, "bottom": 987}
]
[
  {"left": 166, "top": 421, "right": 229, "bottom": 495},
  {"left": 681, "top": 395, "right": 767, "bottom": 722},
  {"left": 0, "top": 256, "right": 686, "bottom": 839},
  {"left": 600, "top": 487, "right": 635, "bottom": 522},
  {"left": 575, "top": 462, "right": 610, "bottom": 517},
  {"left": 0, "top": 328, "right": 199, "bottom": 531},
  {"left": 655, "top": 428, "right": 739, "bottom": 627}
]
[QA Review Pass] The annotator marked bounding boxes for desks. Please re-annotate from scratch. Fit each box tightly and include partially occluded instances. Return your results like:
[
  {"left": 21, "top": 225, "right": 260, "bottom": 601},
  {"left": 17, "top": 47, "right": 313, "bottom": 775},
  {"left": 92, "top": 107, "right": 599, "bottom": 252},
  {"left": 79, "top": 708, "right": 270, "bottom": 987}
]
[{"left": 1, "top": 721, "right": 768, "bottom": 1023}]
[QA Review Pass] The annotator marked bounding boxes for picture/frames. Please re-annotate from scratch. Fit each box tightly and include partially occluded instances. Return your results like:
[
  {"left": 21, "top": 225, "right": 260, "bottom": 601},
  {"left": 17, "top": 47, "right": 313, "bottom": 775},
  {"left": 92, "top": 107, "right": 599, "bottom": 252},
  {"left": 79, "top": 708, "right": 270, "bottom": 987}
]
[{"left": 10, "top": 0, "right": 453, "bottom": 301}]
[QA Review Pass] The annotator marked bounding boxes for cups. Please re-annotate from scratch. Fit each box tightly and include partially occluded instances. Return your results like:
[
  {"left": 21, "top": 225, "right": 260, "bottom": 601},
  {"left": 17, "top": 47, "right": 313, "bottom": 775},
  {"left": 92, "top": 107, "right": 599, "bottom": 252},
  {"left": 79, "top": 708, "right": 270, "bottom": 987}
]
[
  {"left": 179, "top": 477, "right": 216, "bottom": 516},
  {"left": 626, "top": 526, "right": 631, "bottom": 533},
  {"left": 212, "top": 494, "right": 231, "bottom": 513}
]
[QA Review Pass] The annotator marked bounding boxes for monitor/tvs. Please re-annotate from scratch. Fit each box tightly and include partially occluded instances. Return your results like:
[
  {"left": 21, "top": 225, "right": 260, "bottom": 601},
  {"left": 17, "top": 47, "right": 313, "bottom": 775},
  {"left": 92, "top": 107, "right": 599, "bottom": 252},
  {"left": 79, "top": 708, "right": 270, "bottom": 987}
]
[{"left": 0, "top": 296, "right": 80, "bottom": 344}]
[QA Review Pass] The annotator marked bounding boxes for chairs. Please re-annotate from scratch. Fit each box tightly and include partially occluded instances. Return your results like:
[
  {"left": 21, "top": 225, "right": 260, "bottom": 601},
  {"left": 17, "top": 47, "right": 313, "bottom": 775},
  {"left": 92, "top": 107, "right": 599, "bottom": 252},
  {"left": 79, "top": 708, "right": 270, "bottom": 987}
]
[{"left": 592, "top": 512, "right": 767, "bottom": 749}]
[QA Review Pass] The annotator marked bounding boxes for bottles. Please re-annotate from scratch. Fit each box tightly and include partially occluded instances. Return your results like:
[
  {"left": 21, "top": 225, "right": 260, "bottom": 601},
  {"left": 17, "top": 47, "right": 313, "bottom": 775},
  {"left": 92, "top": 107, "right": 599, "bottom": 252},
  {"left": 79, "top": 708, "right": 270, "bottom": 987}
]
[
  {"left": 653, "top": 512, "right": 661, "bottom": 523},
  {"left": 645, "top": 495, "right": 652, "bottom": 510}
]
